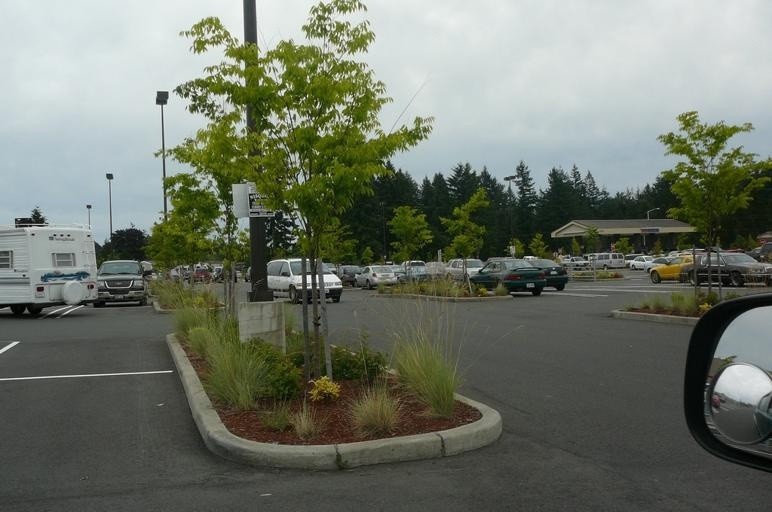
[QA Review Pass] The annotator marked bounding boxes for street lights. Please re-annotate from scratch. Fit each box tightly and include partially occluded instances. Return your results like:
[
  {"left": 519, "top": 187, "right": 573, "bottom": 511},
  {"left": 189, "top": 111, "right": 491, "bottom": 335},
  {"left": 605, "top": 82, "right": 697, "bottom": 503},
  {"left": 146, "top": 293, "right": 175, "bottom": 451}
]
[
  {"left": 105, "top": 173, "right": 114, "bottom": 239},
  {"left": 504, "top": 173, "right": 516, "bottom": 242},
  {"left": 86, "top": 205, "right": 92, "bottom": 229},
  {"left": 646, "top": 206, "right": 660, "bottom": 223},
  {"left": 153, "top": 88, "right": 172, "bottom": 226}
]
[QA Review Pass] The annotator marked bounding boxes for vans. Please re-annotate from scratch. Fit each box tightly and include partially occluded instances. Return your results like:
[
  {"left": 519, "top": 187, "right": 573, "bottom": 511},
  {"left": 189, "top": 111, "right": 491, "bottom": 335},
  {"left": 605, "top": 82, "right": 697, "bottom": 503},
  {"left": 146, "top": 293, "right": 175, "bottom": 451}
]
[{"left": 265, "top": 258, "right": 343, "bottom": 304}]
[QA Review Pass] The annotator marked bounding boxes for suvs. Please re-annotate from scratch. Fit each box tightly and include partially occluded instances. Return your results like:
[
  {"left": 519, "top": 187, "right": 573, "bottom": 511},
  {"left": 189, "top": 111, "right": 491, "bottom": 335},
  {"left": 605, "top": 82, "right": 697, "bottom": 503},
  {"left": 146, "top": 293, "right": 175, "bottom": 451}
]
[{"left": 92, "top": 260, "right": 147, "bottom": 307}]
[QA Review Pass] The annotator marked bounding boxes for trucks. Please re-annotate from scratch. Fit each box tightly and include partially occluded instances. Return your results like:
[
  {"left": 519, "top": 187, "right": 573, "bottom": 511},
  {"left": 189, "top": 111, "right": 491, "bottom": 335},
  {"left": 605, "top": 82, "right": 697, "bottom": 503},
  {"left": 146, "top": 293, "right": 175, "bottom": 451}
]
[{"left": 0, "top": 216, "right": 98, "bottom": 314}]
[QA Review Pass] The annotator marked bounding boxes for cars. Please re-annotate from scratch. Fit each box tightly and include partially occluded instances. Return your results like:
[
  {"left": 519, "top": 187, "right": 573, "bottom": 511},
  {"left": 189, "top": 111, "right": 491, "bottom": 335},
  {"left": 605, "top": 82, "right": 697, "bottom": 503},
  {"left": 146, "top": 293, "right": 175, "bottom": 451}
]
[
  {"left": 711, "top": 393, "right": 728, "bottom": 408},
  {"left": 141, "top": 261, "right": 253, "bottom": 285},
  {"left": 752, "top": 390, "right": 771, "bottom": 438}
]
